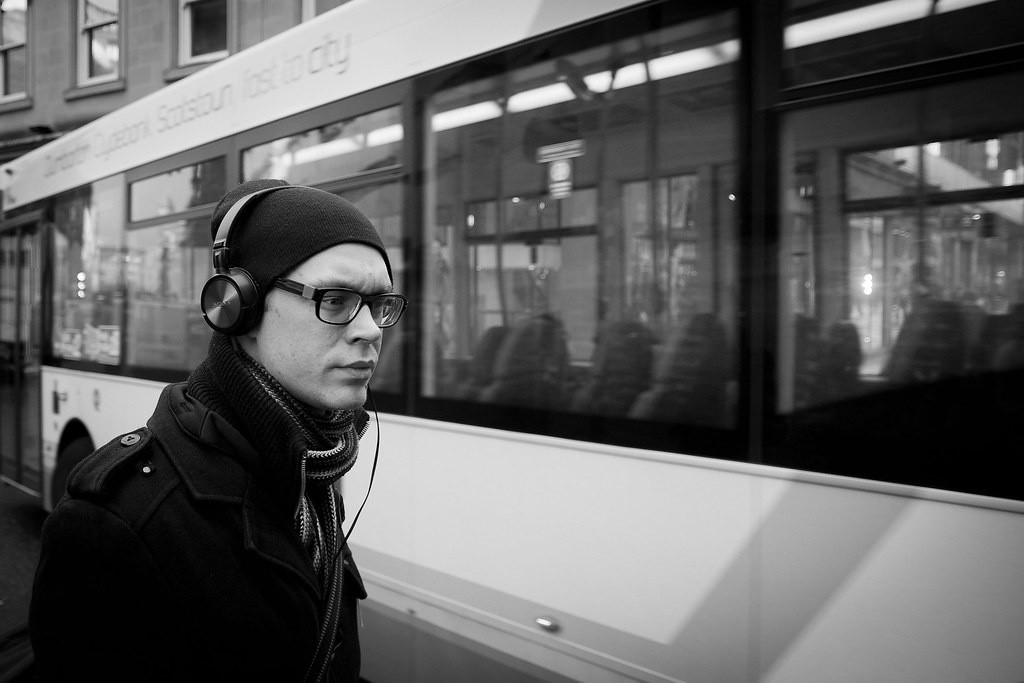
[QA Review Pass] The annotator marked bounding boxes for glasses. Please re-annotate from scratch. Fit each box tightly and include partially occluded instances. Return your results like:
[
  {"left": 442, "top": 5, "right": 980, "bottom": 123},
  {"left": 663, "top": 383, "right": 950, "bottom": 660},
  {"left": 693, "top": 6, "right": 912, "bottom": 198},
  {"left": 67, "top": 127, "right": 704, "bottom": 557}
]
[{"left": 271, "top": 277, "right": 407, "bottom": 329}]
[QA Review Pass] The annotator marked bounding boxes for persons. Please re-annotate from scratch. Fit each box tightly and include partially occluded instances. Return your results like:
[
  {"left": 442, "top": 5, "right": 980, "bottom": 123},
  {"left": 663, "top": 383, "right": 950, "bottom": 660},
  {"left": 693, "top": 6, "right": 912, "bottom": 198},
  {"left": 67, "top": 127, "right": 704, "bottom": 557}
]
[{"left": 27, "top": 178, "right": 394, "bottom": 683}]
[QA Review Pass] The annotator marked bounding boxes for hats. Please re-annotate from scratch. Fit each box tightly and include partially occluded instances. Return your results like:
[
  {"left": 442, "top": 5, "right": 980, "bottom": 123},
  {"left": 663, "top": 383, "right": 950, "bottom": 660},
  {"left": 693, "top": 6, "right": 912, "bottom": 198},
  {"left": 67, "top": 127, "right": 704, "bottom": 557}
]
[{"left": 210, "top": 179, "right": 393, "bottom": 304}]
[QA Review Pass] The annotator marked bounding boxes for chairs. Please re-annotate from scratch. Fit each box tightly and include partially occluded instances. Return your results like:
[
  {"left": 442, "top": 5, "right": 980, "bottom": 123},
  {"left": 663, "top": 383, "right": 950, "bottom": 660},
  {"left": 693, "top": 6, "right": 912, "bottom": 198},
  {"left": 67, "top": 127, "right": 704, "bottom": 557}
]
[
  {"left": 879, "top": 296, "right": 982, "bottom": 390},
  {"left": 577, "top": 321, "right": 654, "bottom": 414},
  {"left": 455, "top": 326, "right": 509, "bottom": 401},
  {"left": 481, "top": 312, "right": 574, "bottom": 409},
  {"left": 634, "top": 312, "right": 726, "bottom": 419},
  {"left": 813, "top": 322, "right": 864, "bottom": 408},
  {"left": 369, "top": 337, "right": 442, "bottom": 393}
]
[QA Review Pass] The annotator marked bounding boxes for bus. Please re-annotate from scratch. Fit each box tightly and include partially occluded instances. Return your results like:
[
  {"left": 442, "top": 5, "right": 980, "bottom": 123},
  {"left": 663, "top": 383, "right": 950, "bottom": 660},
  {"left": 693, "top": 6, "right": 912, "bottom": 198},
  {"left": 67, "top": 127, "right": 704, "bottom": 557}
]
[{"left": 0, "top": 1, "right": 1024, "bottom": 683}]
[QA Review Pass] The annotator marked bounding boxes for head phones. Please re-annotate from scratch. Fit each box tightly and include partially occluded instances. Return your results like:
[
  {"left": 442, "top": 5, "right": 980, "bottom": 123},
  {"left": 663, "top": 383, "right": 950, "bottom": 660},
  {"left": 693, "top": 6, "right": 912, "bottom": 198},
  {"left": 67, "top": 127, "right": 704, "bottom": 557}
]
[{"left": 202, "top": 182, "right": 325, "bottom": 338}]
